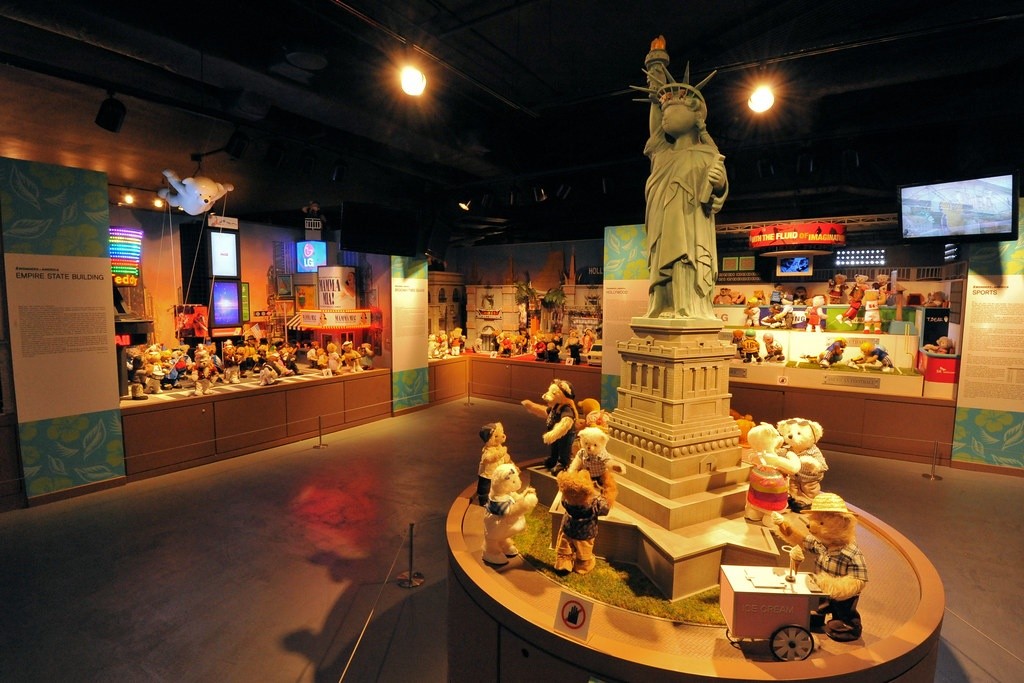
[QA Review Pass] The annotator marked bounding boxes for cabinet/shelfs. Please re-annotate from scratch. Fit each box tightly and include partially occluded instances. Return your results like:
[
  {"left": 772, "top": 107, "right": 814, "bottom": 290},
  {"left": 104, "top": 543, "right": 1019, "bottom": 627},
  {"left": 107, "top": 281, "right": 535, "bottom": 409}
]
[
  {"left": 0, "top": 425, "right": 22, "bottom": 499},
  {"left": 554, "top": 365, "right": 602, "bottom": 415},
  {"left": 428, "top": 359, "right": 468, "bottom": 403},
  {"left": 123, "top": 390, "right": 287, "bottom": 475},
  {"left": 469, "top": 358, "right": 555, "bottom": 407},
  {"left": 729, "top": 385, "right": 785, "bottom": 450},
  {"left": 286, "top": 370, "right": 392, "bottom": 437},
  {"left": 784, "top": 389, "right": 956, "bottom": 462}
]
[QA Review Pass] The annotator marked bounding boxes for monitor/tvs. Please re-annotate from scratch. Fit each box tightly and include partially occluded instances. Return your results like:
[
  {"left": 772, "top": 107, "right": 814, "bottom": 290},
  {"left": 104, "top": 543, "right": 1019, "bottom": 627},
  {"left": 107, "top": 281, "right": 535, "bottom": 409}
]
[
  {"left": 776, "top": 255, "right": 813, "bottom": 276},
  {"left": 296, "top": 240, "right": 328, "bottom": 273},
  {"left": 898, "top": 169, "right": 1021, "bottom": 246}
]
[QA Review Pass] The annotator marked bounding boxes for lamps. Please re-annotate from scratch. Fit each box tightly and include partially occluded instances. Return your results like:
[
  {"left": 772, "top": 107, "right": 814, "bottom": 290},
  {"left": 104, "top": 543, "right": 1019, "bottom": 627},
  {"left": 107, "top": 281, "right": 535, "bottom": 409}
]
[
  {"left": 94, "top": 90, "right": 125, "bottom": 132},
  {"left": 191, "top": 129, "right": 249, "bottom": 160},
  {"left": 329, "top": 164, "right": 347, "bottom": 185},
  {"left": 458, "top": 175, "right": 614, "bottom": 211}
]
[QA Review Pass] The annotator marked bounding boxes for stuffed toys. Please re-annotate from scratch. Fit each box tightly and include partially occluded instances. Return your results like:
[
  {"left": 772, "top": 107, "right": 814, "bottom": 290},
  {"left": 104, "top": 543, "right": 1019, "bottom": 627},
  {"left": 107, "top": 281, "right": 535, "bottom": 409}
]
[
  {"left": 477, "top": 379, "right": 626, "bottom": 574},
  {"left": 491, "top": 328, "right": 596, "bottom": 364},
  {"left": 744, "top": 417, "right": 869, "bottom": 639},
  {"left": 747, "top": 290, "right": 767, "bottom": 305},
  {"left": 429, "top": 328, "right": 483, "bottom": 360},
  {"left": 307, "top": 341, "right": 374, "bottom": 375},
  {"left": 731, "top": 330, "right": 746, "bottom": 358},
  {"left": 713, "top": 288, "right": 744, "bottom": 305},
  {"left": 923, "top": 336, "right": 953, "bottom": 354},
  {"left": 849, "top": 274, "right": 890, "bottom": 303},
  {"left": 922, "top": 291, "right": 945, "bottom": 306},
  {"left": 828, "top": 274, "right": 849, "bottom": 304},
  {"left": 125, "top": 335, "right": 303, "bottom": 397}
]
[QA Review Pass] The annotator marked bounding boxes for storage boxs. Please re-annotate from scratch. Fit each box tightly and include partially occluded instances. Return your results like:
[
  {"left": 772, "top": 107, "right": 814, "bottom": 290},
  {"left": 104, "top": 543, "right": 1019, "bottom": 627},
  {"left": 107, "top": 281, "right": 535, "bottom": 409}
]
[{"left": 917, "top": 346, "right": 961, "bottom": 384}]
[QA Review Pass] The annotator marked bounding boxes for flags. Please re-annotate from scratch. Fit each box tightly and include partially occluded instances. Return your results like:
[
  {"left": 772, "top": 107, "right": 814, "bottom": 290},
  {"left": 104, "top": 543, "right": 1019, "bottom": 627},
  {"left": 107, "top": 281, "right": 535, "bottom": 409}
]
[{"left": 251, "top": 324, "right": 261, "bottom": 338}]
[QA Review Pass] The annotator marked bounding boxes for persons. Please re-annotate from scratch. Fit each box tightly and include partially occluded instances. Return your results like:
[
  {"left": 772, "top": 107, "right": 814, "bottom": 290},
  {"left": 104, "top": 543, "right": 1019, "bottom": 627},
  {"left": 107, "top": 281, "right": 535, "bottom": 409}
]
[
  {"left": 630, "top": 65, "right": 730, "bottom": 319},
  {"left": 742, "top": 282, "right": 894, "bottom": 373}
]
